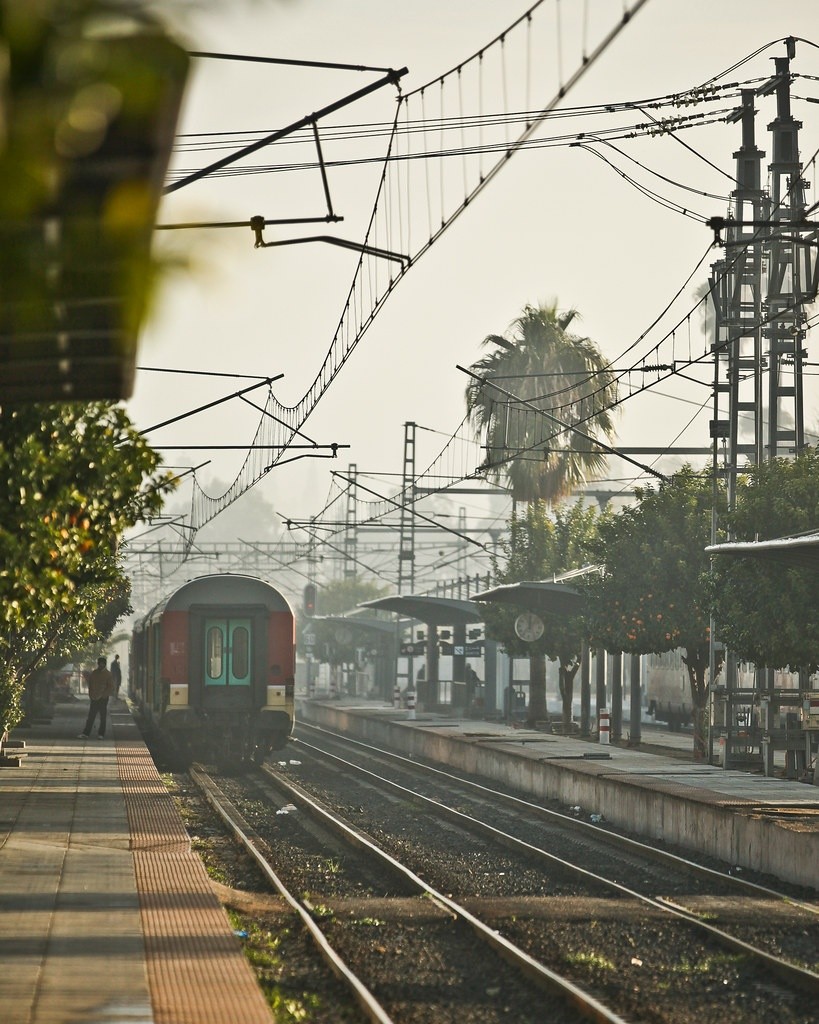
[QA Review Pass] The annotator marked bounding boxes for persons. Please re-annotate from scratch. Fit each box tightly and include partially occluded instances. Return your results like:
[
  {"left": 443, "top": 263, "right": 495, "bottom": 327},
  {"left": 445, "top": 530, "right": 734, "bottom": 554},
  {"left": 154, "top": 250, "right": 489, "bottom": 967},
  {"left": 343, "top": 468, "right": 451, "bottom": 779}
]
[
  {"left": 464, "top": 663, "right": 480, "bottom": 703},
  {"left": 418, "top": 664, "right": 425, "bottom": 679},
  {"left": 111, "top": 654, "right": 121, "bottom": 699},
  {"left": 79, "top": 658, "right": 112, "bottom": 740}
]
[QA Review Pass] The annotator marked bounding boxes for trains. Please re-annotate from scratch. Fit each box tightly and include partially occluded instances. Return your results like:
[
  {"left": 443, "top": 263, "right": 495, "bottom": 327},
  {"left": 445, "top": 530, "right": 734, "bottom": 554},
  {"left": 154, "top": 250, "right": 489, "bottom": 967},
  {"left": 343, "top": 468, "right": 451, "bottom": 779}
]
[{"left": 128, "top": 572, "right": 295, "bottom": 741}]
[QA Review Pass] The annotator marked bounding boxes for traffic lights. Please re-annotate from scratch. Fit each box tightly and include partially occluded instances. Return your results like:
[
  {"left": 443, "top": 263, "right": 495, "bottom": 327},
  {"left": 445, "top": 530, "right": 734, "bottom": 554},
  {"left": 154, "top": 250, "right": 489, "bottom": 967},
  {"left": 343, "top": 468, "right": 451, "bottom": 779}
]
[{"left": 304, "top": 584, "right": 316, "bottom": 615}]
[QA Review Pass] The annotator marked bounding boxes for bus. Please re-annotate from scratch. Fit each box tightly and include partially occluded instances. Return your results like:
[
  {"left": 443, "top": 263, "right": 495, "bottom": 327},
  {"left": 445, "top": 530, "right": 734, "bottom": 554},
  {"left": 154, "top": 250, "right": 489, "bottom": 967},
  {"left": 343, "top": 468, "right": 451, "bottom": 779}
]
[{"left": 645, "top": 637, "right": 741, "bottom": 732}]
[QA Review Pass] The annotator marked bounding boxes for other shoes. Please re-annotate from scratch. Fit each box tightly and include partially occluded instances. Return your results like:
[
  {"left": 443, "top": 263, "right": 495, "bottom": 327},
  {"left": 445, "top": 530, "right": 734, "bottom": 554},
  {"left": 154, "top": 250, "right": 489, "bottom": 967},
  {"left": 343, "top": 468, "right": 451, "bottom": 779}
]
[
  {"left": 98, "top": 735, "right": 103, "bottom": 739},
  {"left": 77, "top": 733, "right": 89, "bottom": 738}
]
